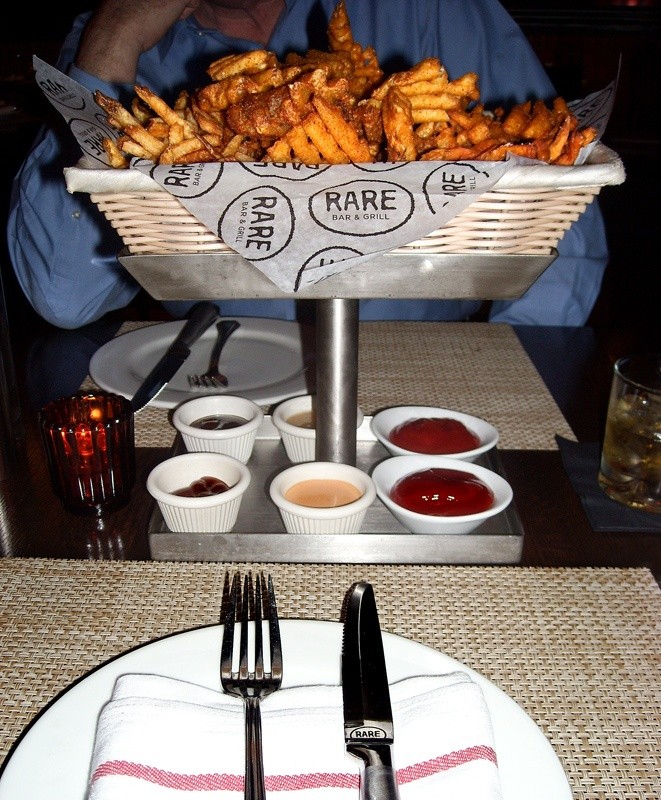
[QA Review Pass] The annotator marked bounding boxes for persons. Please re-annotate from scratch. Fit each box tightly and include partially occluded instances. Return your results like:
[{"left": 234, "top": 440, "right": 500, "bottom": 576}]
[{"left": 6, "top": 0, "right": 610, "bottom": 410}]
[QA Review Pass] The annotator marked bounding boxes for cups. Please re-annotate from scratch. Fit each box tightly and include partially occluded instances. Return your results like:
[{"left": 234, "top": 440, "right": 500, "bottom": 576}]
[
  {"left": 597, "top": 355, "right": 661, "bottom": 515},
  {"left": 37, "top": 389, "right": 136, "bottom": 518}
]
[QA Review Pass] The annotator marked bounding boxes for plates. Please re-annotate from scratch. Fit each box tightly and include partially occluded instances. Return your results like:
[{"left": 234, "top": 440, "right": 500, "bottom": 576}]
[
  {"left": 0, "top": 617, "right": 575, "bottom": 800},
  {"left": 89, "top": 317, "right": 316, "bottom": 409}
]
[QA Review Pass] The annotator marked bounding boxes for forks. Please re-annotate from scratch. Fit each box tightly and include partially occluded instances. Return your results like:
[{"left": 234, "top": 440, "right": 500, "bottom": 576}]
[
  {"left": 219, "top": 572, "right": 284, "bottom": 800},
  {"left": 189, "top": 320, "right": 240, "bottom": 388}
]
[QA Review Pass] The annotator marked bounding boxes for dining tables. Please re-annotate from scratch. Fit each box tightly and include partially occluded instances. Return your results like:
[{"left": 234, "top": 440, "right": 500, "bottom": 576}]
[{"left": 0, "top": 311, "right": 661, "bottom": 800}]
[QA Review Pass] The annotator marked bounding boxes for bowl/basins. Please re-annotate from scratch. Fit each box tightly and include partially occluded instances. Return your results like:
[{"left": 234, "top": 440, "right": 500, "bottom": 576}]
[
  {"left": 272, "top": 394, "right": 364, "bottom": 466},
  {"left": 371, "top": 405, "right": 500, "bottom": 463},
  {"left": 268, "top": 460, "right": 377, "bottom": 534},
  {"left": 371, "top": 452, "right": 513, "bottom": 535},
  {"left": 172, "top": 395, "right": 264, "bottom": 465},
  {"left": 145, "top": 451, "right": 252, "bottom": 534}
]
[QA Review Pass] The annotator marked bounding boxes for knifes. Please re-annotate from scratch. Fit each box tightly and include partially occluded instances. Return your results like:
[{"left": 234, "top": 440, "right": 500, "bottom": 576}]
[
  {"left": 130, "top": 300, "right": 222, "bottom": 413},
  {"left": 340, "top": 583, "right": 400, "bottom": 800}
]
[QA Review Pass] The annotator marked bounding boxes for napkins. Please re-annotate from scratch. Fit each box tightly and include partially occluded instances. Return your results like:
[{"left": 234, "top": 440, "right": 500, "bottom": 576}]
[{"left": 87, "top": 669, "right": 502, "bottom": 800}]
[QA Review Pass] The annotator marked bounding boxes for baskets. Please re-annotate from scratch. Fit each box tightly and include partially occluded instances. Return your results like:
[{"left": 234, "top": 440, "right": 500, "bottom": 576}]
[{"left": 64, "top": 142, "right": 625, "bottom": 257}]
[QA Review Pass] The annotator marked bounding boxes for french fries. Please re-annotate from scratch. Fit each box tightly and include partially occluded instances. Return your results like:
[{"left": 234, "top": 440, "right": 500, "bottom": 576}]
[{"left": 94, "top": 0, "right": 599, "bottom": 168}]
